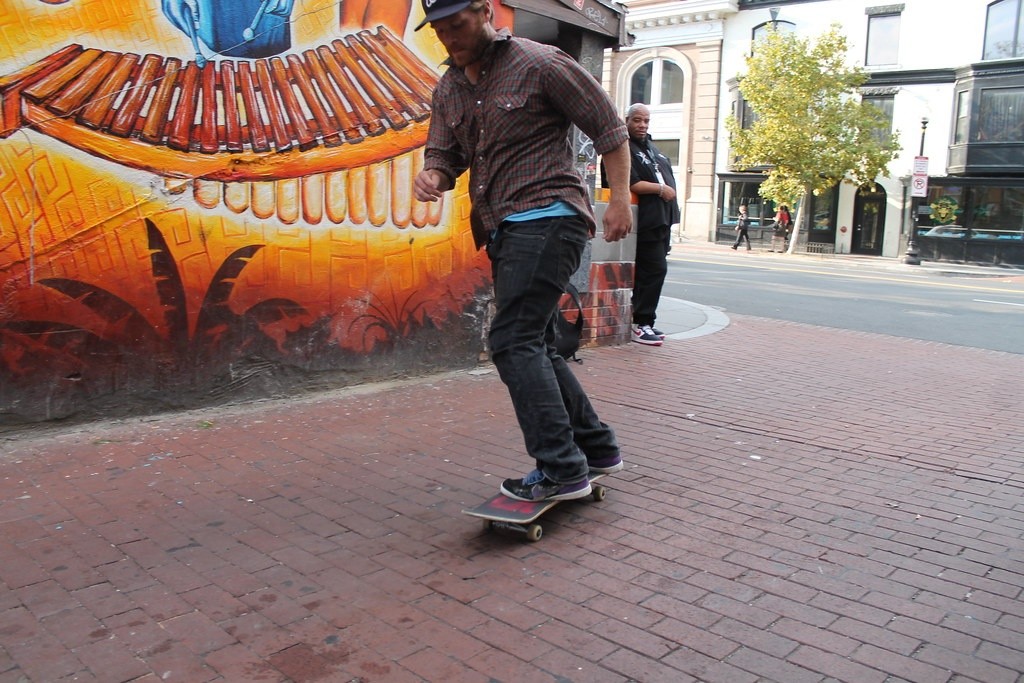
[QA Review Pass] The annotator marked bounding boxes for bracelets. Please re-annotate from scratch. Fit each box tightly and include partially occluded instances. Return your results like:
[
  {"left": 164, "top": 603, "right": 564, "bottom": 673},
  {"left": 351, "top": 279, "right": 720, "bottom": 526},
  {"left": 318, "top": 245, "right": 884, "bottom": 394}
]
[{"left": 659, "top": 182, "right": 664, "bottom": 197}]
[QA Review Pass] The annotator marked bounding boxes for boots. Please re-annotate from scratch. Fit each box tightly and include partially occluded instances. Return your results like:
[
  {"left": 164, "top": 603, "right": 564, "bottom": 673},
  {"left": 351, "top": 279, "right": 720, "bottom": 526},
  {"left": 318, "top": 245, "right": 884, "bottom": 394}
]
[
  {"left": 746, "top": 241, "right": 751, "bottom": 250},
  {"left": 730, "top": 241, "right": 740, "bottom": 250}
]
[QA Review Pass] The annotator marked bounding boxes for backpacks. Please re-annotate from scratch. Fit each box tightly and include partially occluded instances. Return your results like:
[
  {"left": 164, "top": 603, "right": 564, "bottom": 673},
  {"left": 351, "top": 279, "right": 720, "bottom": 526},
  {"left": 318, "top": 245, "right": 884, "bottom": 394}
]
[{"left": 552, "top": 283, "right": 584, "bottom": 360}]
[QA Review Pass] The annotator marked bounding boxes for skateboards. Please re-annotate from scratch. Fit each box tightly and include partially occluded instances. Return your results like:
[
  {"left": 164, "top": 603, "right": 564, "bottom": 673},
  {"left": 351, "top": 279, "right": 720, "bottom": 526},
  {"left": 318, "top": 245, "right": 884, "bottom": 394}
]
[{"left": 461, "top": 467, "right": 608, "bottom": 541}]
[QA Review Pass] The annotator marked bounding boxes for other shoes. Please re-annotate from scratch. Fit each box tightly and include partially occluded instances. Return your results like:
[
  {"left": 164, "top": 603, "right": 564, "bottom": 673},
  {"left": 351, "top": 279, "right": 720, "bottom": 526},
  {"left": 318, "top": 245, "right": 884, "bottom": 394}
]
[
  {"left": 778, "top": 250, "right": 783, "bottom": 253},
  {"left": 768, "top": 249, "right": 774, "bottom": 252}
]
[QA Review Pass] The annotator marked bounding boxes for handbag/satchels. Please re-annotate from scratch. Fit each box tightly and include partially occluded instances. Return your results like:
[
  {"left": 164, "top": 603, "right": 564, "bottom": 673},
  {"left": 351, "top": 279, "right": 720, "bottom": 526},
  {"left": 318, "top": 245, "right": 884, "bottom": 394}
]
[
  {"left": 787, "top": 222, "right": 794, "bottom": 234},
  {"left": 739, "top": 219, "right": 750, "bottom": 227}
]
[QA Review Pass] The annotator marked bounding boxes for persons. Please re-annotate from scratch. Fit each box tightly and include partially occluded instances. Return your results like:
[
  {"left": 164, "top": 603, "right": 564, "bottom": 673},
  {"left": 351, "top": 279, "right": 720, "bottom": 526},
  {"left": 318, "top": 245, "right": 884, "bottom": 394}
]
[
  {"left": 769, "top": 205, "right": 792, "bottom": 253},
  {"left": 414, "top": 0, "right": 634, "bottom": 502},
  {"left": 600, "top": 103, "right": 680, "bottom": 346},
  {"left": 731, "top": 205, "right": 752, "bottom": 250}
]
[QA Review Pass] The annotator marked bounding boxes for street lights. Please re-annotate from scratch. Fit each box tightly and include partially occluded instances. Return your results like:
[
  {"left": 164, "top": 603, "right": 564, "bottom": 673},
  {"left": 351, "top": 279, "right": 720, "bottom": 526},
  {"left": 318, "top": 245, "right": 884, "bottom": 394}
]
[{"left": 904, "top": 117, "right": 929, "bottom": 265}]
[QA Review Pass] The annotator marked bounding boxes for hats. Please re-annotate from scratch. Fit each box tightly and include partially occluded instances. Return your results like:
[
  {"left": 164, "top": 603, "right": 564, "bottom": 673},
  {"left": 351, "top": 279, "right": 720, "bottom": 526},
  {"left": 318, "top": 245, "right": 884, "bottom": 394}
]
[{"left": 414, "top": 0, "right": 471, "bottom": 32}]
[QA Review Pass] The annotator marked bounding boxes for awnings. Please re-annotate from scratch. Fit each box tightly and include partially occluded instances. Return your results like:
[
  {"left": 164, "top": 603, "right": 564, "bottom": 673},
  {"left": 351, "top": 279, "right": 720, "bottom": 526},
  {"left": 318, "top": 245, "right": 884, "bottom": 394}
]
[{"left": 716, "top": 172, "right": 769, "bottom": 182}]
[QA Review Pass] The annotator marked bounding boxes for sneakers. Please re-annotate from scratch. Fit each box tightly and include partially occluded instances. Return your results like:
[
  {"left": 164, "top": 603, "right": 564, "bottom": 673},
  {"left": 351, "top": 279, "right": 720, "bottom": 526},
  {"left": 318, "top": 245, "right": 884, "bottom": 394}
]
[
  {"left": 587, "top": 453, "right": 623, "bottom": 474},
  {"left": 500, "top": 469, "right": 592, "bottom": 502},
  {"left": 630, "top": 323, "right": 663, "bottom": 346},
  {"left": 652, "top": 326, "right": 665, "bottom": 339}
]
